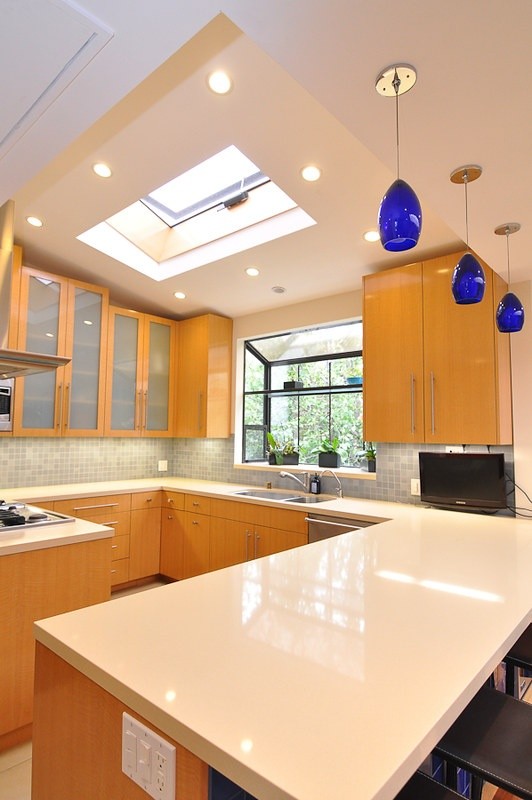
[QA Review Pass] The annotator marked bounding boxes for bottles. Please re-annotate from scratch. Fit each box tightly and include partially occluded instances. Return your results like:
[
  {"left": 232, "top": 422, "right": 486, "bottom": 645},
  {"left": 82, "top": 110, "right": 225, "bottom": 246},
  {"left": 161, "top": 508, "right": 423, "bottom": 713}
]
[{"left": 309, "top": 472, "right": 320, "bottom": 494}]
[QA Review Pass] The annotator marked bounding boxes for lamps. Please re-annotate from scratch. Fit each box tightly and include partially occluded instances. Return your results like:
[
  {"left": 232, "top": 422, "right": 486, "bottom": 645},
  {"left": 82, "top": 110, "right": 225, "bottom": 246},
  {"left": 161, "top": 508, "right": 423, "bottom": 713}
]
[
  {"left": 491, "top": 221, "right": 524, "bottom": 333},
  {"left": 374, "top": 61, "right": 423, "bottom": 251},
  {"left": 448, "top": 165, "right": 485, "bottom": 306}
]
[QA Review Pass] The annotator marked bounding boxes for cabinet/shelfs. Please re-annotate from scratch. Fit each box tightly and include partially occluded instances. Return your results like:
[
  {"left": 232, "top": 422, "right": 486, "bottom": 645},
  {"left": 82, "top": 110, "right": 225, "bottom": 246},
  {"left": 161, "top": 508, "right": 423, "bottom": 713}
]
[
  {"left": 129, "top": 490, "right": 162, "bottom": 580},
  {"left": 210, "top": 498, "right": 309, "bottom": 571},
  {"left": 0, "top": 245, "right": 233, "bottom": 438},
  {"left": 360, "top": 251, "right": 513, "bottom": 446},
  {"left": 53, "top": 494, "right": 131, "bottom": 592},
  {"left": 159, "top": 491, "right": 211, "bottom": 581}
]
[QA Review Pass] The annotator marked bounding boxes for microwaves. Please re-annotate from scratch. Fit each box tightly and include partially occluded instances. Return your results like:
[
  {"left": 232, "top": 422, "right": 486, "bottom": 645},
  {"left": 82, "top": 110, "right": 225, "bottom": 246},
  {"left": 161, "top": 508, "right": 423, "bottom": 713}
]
[{"left": 0, "top": 378, "right": 15, "bottom": 432}]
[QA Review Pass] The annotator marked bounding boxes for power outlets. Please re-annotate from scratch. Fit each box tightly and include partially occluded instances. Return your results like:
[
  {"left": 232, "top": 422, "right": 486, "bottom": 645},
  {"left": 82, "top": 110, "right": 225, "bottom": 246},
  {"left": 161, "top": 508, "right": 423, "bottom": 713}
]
[{"left": 119, "top": 713, "right": 175, "bottom": 800}]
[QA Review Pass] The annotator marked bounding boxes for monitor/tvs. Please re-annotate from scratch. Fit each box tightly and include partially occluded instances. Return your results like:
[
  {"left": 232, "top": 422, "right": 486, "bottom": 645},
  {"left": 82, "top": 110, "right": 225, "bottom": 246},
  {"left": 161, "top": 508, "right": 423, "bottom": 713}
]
[{"left": 419, "top": 452, "right": 508, "bottom": 511}]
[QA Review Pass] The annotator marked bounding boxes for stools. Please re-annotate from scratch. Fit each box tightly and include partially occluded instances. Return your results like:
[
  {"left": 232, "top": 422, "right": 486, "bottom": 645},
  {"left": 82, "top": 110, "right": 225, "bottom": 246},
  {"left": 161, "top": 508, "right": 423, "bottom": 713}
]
[
  {"left": 429, "top": 685, "right": 532, "bottom": 800},
  {"left": 502, "top": 621, "right": 532, "bottom": 698},
  {"left": 394, "top": 769, "right": 470, "bottom": 800}
]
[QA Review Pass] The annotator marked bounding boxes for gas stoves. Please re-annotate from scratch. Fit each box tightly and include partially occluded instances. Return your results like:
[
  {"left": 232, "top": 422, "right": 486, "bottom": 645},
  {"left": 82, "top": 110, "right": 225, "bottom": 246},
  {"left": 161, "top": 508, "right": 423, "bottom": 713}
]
[{"left": 0, "top": 499, "right": 75, "bottom": 532}]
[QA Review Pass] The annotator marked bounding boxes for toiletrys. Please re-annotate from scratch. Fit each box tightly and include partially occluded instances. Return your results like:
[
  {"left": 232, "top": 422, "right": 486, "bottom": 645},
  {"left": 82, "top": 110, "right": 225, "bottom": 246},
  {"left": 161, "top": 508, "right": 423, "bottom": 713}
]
[{"left": 310, "top": 472, "right": 321, "bottom": 494}]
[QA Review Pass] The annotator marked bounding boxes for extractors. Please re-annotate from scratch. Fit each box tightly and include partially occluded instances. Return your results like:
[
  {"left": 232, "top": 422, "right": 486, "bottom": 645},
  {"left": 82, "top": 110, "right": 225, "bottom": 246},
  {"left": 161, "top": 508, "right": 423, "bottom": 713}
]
[{"left": 0, "top": 200, "right": 72, "bottom": 380}]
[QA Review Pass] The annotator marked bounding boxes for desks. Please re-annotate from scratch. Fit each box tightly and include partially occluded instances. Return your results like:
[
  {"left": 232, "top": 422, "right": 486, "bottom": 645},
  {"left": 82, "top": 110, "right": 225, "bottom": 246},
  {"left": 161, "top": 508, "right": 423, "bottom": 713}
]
[{"left": 32, "top": 508, "right": 532, "bottom": 800}]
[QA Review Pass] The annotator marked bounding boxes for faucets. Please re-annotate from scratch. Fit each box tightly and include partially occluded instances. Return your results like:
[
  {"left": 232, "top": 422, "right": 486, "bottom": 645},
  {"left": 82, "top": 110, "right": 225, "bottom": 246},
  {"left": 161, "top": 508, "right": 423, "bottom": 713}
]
[
  {"left": 319, "top": 469, "right": 343, "bottom": 499},
  {"left": 279, "top": 471, "right": 310, "bottom": 493}
]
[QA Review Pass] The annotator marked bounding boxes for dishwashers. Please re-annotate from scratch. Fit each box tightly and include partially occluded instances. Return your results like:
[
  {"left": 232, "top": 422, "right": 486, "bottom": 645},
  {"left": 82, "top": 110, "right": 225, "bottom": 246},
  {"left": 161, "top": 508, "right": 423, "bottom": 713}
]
[{"left": 304, "top": 513, "right": 379, "bottom": 544}]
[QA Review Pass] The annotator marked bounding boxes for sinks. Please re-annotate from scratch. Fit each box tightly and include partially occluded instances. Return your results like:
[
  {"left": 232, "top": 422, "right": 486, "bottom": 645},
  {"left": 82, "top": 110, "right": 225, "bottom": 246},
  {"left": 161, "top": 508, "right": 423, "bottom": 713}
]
[
  {"left": 235, "top": 490, "right": 303, "bottom": 501},
  {"left": 282, "top": 497, "right": 336, "bottom": 504}
]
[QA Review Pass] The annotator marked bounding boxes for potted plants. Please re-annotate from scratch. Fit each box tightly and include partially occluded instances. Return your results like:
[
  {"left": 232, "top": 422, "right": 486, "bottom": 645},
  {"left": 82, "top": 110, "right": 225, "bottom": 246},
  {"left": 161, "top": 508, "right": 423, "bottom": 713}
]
[
  {"left": 311, "top": 437, "right": 341, "bottom": 469},
  {"left": 344, "top": 367, "right": 363, "bottom": 384},
  {"left": 284, "top": 367, "right": 303, "bottom": 390},
  {"left": 355, "top": 441, "right": 376, "bottom": 472},
  {"left": 265, "top": 431, "right": 307, "bottom": 466}
]
